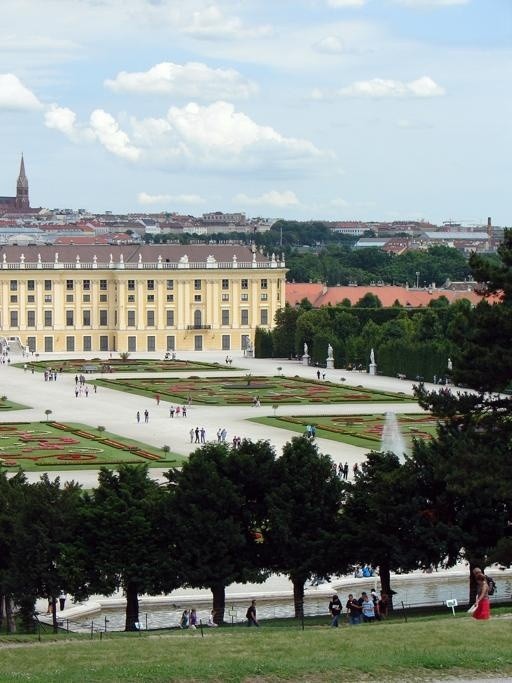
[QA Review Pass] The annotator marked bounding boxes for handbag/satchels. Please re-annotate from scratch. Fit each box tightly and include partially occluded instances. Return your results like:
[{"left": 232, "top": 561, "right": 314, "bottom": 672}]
[{"left": 467, "top": 603, "right": 478, "bottom": 613}]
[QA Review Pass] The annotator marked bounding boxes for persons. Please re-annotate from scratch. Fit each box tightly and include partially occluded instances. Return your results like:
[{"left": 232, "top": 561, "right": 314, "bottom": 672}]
[
  {"left": 228, "top": 356, "right": 232, "bottom": 366},
  {"left": 471, "top": 567, "right": 490, "bottom": 597},
  {"left": 317, "top": 370, "right": 321, "bottom": 379},
  {"left": 225, "top": 356, "right": 228, "bottom": 363},
  {"left": 330, "top": 461, "right": 359, "bottom": 480},
  {"left": 188, "top": 609, "right": 196, "bottom": 630},
  {"left": 304, "top": 343, "right": 310, "bottom": 356},
  {"left": 447, "top": 357, "right": 452, "bottom": 370},
  {"left": 246, "top": 600, "right": 259, "bottom": 628},
  {"left": 310, "top": 425, "right": 315, "bottom": 440},
  {"left": 322, "top": 370, "right": 326, "bottom": 380},
  {"left": 472, "top": 574, "right": 490, "bottom": 621},
  {"left": 254, "top": 396, "right": 261, "bottom": 407},
  {"left": 2, "top": 341, "right": 97, "bottom": 399},
  {"left": 207, "top": 610, "right": 219, "bottom": 627},
  {"left": 180, "top": 610, "right": 189, "bottom": 628},
  {"left": 306, "top": 424, "right": 312, "bottom": 439},
  {"left": 327, "top": 343, "right": 334, "bottom": 359},
  {"left": 164, "top": 352, "right": 177, "bottom": 360},
  {"left": 103, "top": 364, "right": 112, "bottom": 373},
  {"left": 328, "top": 562, "right": 390, "bottom": 626},
  {"left": 252, "top": 395, "right": 257, "bottom": 407},
  {"left": 135, "top": 391, "right": 244, "bottom": 448},
  {"left": 370, "top": 348, "right": 375, "bottom": 363},
  {"left": 358, "top": 362, "right": 363, "bottom": 373}
]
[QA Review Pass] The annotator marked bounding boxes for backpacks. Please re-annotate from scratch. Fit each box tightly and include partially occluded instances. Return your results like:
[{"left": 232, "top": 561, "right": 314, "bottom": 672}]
[{"left": 486, "top": 574, "right": 497, "bottom": 595}]
